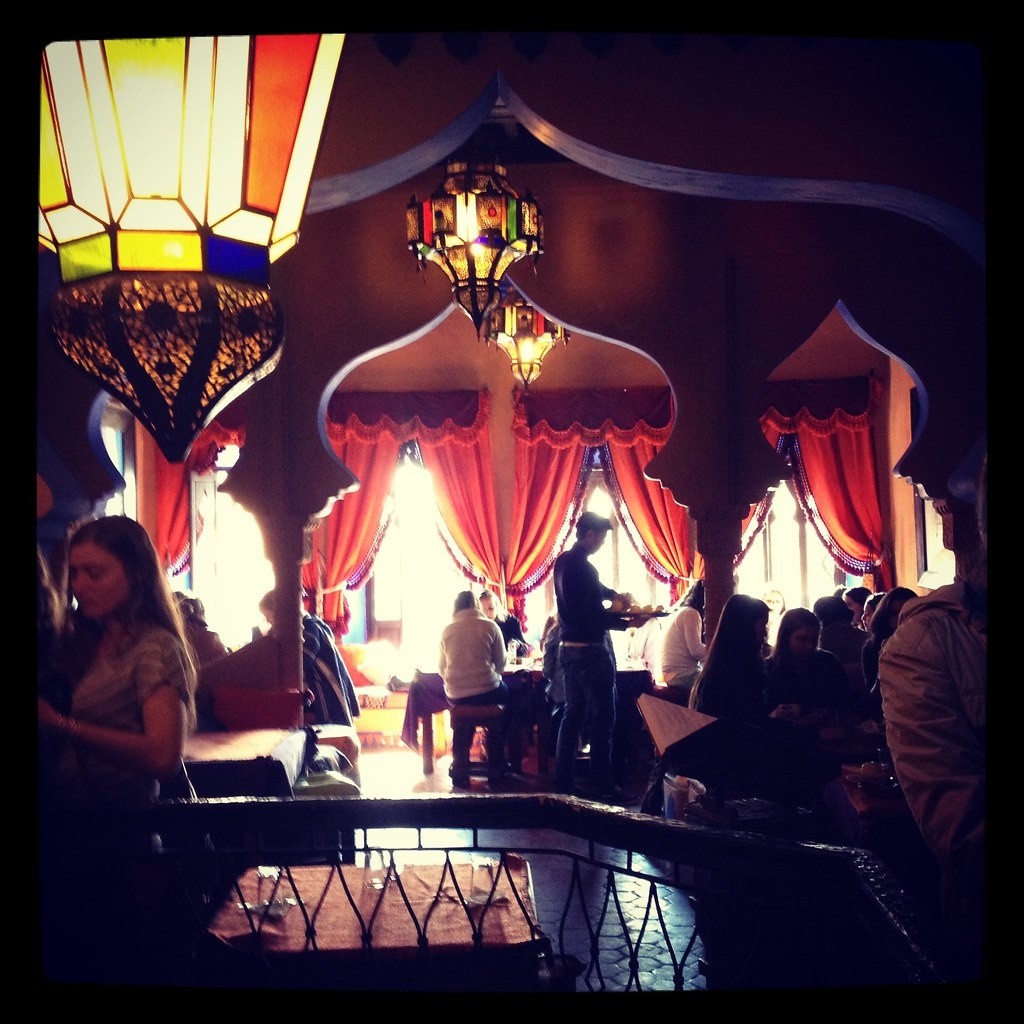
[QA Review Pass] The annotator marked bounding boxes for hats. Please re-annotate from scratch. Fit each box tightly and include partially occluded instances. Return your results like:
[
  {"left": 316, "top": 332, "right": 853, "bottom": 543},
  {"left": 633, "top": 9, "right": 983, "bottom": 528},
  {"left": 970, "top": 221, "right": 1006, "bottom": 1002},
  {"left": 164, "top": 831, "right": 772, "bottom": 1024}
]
[{"left": 575, "top": 512, "right": 613, "bottom": 531}]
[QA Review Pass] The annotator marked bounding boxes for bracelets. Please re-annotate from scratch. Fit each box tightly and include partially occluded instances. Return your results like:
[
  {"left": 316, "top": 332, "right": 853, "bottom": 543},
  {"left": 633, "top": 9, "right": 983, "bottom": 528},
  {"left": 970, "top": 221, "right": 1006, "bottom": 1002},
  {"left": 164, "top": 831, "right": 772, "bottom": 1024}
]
[{"left": 55, "top": 712, "right": 83, "bottom": 740}]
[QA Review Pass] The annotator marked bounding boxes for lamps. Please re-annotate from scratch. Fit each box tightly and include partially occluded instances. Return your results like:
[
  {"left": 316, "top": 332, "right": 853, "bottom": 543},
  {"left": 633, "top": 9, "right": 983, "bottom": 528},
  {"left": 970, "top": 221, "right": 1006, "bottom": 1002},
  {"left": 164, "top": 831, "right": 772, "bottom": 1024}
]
[
  {"left": 483, "top": 287, "right": 570, "bottom": 392},
  {"left": 39, "top": 33, "right": 348, "bottom": 463},
  {"left": 404, "top": 138, "right": 546, "bottom": 342}
]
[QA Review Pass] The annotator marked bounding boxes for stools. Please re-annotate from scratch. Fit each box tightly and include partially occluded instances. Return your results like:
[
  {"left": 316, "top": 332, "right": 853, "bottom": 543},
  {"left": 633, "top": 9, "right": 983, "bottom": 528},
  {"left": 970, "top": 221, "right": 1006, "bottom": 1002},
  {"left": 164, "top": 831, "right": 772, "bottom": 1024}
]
[
  {"left": 310, "top": 722, "right": 360, "bottom": 771},
  {"left": 449, "top": 704, "right": 511, "bottom": 789}
]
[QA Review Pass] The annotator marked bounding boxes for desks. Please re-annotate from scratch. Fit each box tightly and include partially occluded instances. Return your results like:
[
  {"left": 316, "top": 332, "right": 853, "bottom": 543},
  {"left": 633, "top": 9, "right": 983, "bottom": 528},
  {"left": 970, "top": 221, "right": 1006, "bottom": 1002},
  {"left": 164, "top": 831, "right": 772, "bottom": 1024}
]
[
  {"left": 400, "top": 660, "right": 651, "bottom": 775},
  {"left": 183, "top": 727, "right": 309, "bottom": 804},
  {"left": 838, "top": 764, "right": 938, "bottom": 914}
]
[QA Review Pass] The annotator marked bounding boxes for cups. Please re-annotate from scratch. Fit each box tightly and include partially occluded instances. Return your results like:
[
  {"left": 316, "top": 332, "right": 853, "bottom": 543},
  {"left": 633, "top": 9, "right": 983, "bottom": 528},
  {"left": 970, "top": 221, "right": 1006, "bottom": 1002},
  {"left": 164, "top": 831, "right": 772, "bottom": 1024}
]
[
  {"left": 363, "top": 846, "right": 386, "bottom": 890},
  {"left": 469, "top": 857, "right": 496, "bottom": 904},
  {"left": 507, "top": 643, "right": 517, "bottom": 664},
  {"left": 255, "top": 866, "right": 283, "bottom": 908}
]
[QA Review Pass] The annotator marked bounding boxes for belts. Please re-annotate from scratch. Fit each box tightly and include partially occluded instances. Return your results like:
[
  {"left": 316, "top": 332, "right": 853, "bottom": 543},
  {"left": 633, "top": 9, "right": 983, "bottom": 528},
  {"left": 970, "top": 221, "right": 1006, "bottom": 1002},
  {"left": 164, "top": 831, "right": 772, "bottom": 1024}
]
[{"left": 559, "top": 641, "right": 601, "bottom": 648}]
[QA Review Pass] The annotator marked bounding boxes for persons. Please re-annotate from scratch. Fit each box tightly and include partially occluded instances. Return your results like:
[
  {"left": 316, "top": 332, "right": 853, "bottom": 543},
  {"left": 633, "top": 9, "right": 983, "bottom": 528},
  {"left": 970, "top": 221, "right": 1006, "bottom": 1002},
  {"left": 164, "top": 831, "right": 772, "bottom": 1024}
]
[
  {"left": 534, "top": 613, "right": 567, "bottom": 775},
  {"left": 550, "top": 512, "right": 650, "bottom": 805},
  {"left": 437, "top": 590, "right": 513, "bottom": 787},
  {"left": 251, "top": 589, "right": 362, "bottom": 789},
  {"left": 645, "top": 405, "right": 989, "bottom": 877},
  {"left": 179, "top": 598, "right": 234, "bottom": 672},
  {"left": 37, "top": 534, "right": 72, "bottom": 708},
  {"left": 36, "top": 514, "right": 217, "bottom": 984},
  {"left": 479, "top": 589, "right": 529, "bottom": 663}
]
